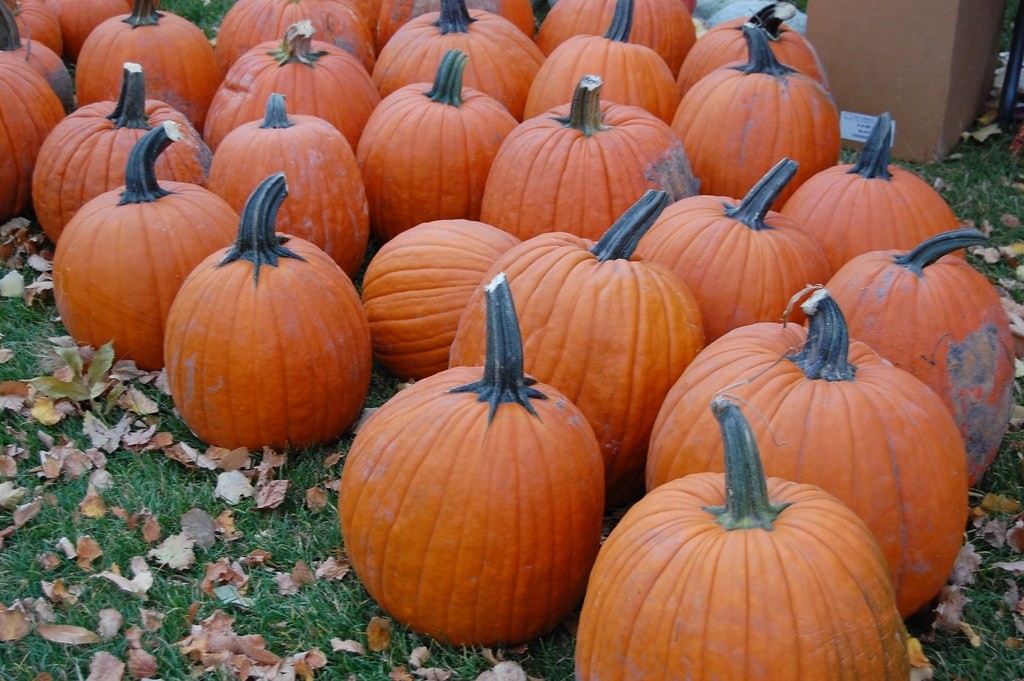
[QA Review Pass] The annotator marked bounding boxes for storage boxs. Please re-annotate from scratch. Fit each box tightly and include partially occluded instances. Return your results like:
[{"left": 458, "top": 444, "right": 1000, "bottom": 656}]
[{"left": 803, "top": 0, "right": 1007, "bottom": 165}]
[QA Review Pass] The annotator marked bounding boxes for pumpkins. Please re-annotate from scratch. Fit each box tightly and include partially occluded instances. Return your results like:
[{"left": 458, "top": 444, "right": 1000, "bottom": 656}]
[{"left": 0, "top": 0, "right": 1016, "bottom": 681}]
